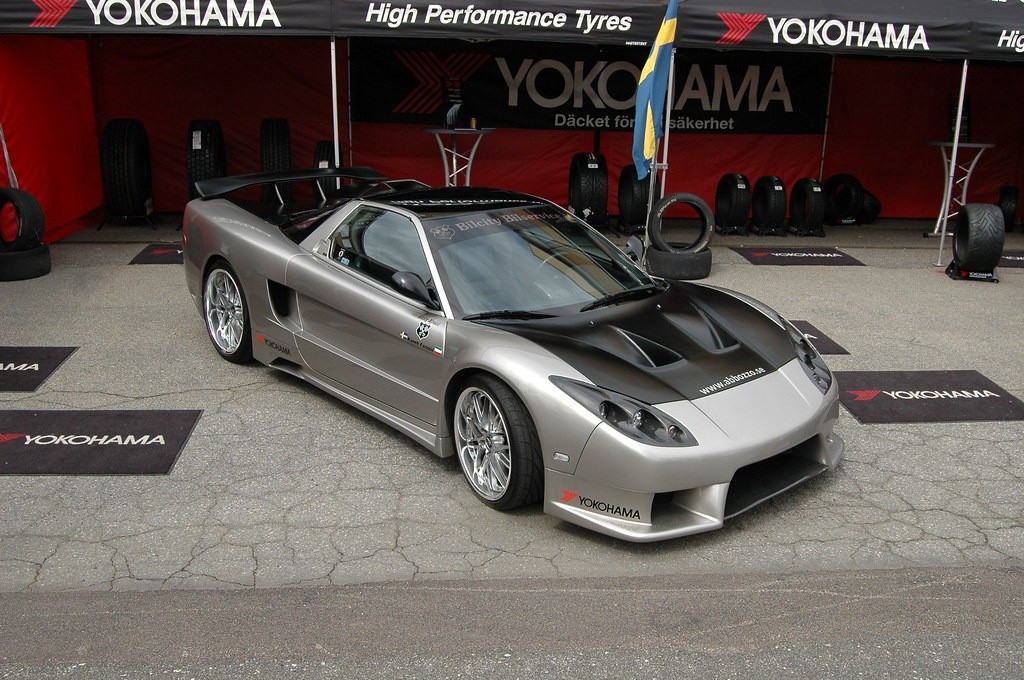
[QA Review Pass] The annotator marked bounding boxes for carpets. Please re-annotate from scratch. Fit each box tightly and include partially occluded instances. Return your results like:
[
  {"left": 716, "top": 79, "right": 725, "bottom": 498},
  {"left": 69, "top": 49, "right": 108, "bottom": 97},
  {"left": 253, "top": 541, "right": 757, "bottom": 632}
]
[
  {"left": 0, "top": 410, "right": 204, "bottom": 476},
  {"left": 831, "top": 368, "right": 1024, "bottom": 425},
  {"left": 127, "top": 243, "right": 184, "bottom": 264},
  {"left": 789, "top": 319, "right": 851, "bottom": 355},
  {"left": 0, "top": 345, "right": 81, "bottom": 392},
  {"left": 729, "top": 246, "right": 867, "bottom": 267}
]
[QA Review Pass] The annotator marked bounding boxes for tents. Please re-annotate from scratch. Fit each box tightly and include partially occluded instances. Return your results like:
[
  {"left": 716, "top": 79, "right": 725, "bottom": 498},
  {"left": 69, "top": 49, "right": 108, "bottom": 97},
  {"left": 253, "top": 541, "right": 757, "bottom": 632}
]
[{"left": 0, "top": 0, "right": 1024, "bottom": 264}]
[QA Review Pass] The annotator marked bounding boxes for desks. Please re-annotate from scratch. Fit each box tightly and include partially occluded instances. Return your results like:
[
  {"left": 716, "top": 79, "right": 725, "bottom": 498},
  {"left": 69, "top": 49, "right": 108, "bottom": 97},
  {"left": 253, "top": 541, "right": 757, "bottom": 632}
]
[
  {"left": 421, "top": 128, "right": 496, "bottom": 187},
  {"left": 923, "top": 139, "right": 996, "bottom": 237}
]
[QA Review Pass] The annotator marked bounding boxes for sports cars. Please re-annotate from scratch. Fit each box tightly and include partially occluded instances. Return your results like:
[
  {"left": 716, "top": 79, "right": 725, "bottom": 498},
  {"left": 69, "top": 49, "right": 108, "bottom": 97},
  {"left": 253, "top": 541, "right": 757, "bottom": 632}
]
[{"left": 181, "top": 166, "right": 848, "bottom": 545}]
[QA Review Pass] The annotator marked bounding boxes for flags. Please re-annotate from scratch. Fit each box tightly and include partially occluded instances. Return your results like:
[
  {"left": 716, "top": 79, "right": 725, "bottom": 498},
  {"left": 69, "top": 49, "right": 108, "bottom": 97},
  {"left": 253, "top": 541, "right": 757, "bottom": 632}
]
[{"left": 633, "top": 0, "right": 679, "bottom": 180}]
